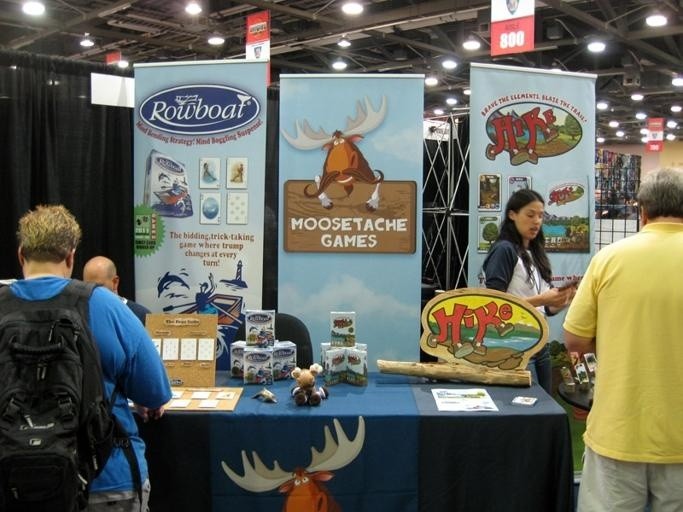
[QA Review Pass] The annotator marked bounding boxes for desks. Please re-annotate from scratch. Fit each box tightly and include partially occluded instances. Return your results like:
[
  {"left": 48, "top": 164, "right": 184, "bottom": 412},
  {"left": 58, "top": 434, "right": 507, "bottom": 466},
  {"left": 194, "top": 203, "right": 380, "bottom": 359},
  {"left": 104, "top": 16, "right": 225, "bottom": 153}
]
[{"left": 128, "top": 368, "right": 568, "bottom": 510}]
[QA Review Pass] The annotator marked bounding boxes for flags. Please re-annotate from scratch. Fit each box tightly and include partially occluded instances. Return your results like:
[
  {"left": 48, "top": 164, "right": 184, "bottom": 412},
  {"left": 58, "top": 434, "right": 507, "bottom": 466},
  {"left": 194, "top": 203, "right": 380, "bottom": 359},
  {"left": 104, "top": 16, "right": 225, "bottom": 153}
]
[
  {"left": 645, "top": 116, "right": 664, "bottom": 155},
  {"left": 488, "top": 0, "right": 536, "bottom": 60},
  {"left": 243, "top": 12, "right": 273, "bottom": 85},
  {"left": 105, "top": 50, "right": 121, "bottom": 66}
]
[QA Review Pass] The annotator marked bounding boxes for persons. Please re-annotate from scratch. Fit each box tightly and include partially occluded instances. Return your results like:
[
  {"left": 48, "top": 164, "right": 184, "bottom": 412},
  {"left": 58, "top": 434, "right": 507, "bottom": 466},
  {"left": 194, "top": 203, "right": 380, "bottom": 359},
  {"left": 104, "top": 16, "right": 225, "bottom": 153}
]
[
  {"left": 82, "top": 255, "right": 153, "bottom": 327},
  {"left": 0, "top": 202, "right": 172, "bottom": 512},
  {"left": 562, "top": 165, "right": 683, "bottom": 511},
  {"left": 481, "top": 189, "right": 579, "bottom": 369}
]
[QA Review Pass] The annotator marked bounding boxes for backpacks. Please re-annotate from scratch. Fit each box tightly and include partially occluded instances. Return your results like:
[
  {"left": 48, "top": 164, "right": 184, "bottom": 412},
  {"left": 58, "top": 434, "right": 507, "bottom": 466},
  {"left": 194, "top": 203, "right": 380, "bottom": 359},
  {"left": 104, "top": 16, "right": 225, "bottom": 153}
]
[{"left": 1, "top": 281, "right": 112, "bottom": 511}]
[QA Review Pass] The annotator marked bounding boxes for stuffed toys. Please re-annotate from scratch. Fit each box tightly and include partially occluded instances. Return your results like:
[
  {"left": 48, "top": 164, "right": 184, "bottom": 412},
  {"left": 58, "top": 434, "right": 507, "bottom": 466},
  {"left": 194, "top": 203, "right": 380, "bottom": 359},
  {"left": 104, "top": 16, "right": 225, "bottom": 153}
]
[{"left": 289, "top": 361, "right": 329, "bottom": 407}]
